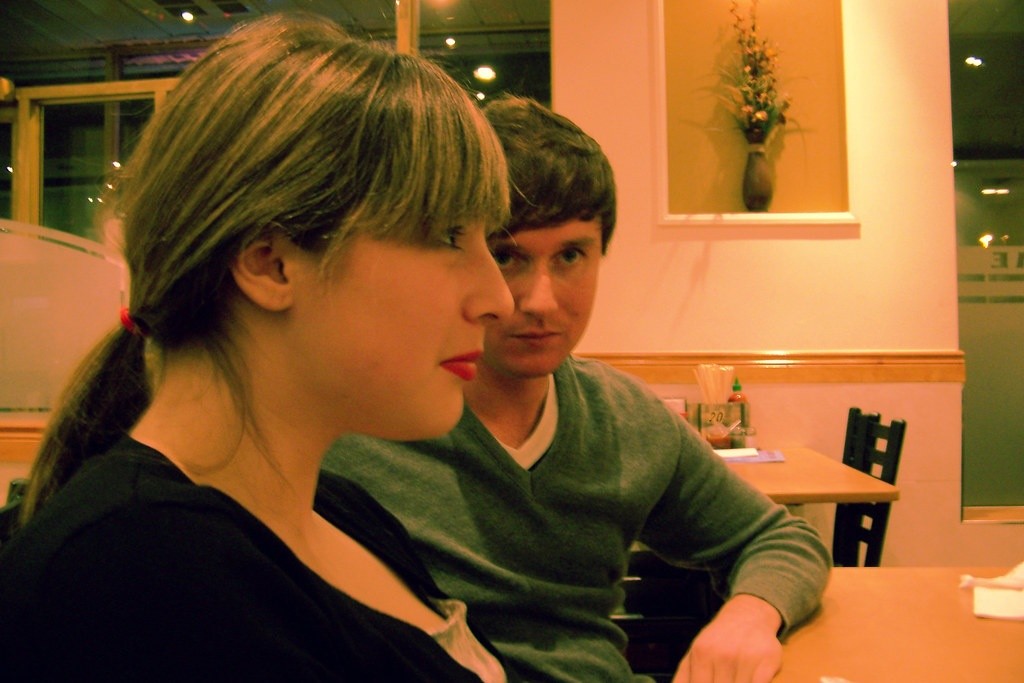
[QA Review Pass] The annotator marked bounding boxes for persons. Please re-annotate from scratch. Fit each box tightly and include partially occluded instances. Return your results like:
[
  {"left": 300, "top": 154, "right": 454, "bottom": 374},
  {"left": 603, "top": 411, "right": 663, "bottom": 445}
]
[
  {"left": 0, "top": 8, "right": 519, "bottom": 683},
  {"left": 320, "top": 93, "right": 836, "bottom": 683}
]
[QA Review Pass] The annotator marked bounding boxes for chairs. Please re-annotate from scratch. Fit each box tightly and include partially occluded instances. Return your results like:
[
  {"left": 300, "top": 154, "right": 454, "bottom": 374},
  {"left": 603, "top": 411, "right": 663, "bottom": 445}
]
[{"left": 830, "top": 407, "right": 906, "bottom": 565}]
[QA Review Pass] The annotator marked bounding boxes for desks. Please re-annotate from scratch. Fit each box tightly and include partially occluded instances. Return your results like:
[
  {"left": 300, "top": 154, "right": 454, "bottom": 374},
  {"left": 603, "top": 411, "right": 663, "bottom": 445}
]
[
  {"left": 771, "top": 567, "right": 1023, "bottom": 682},
  {"left": 726, "top": 445, "right": 901, "bottom": 516}
]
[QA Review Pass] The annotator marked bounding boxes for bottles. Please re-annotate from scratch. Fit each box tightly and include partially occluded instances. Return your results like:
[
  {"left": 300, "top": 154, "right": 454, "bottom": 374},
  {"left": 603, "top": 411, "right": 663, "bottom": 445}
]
[{"left": 728, "top": 377, "right": 748, "bottom": 448}]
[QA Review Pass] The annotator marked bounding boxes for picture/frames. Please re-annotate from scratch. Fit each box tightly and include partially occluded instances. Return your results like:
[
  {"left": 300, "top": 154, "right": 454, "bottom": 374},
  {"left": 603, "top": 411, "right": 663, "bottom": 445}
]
[{"left": 650, "top": 0, "right": 859, "bottom": 226}]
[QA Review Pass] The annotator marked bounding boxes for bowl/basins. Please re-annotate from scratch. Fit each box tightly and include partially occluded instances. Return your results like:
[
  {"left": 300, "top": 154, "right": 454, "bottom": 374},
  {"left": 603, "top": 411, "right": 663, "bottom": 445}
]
[{"left": 701, "top": 427, "right": 733, "bottom": 449}]
